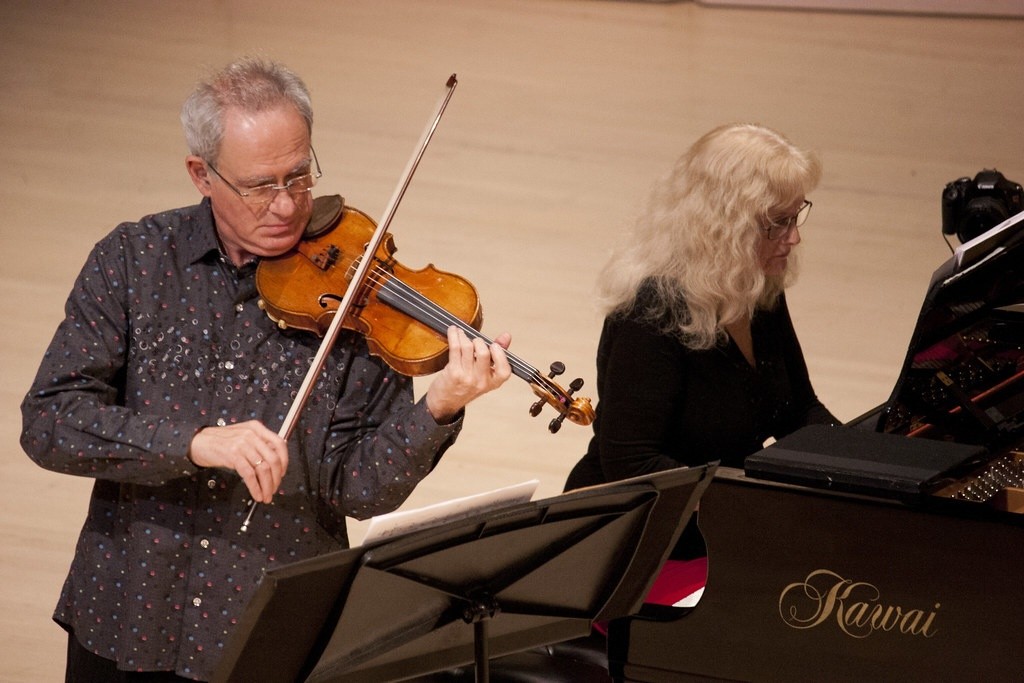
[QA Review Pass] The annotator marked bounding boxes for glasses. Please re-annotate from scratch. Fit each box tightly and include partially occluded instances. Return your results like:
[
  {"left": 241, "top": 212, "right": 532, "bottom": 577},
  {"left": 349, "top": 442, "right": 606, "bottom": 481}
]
[
  {"left": 196, "top": 144, "right": 323, "bottom": 203},
  {"left": 760, "top": 200, "right": 812, "bottom": 239}
]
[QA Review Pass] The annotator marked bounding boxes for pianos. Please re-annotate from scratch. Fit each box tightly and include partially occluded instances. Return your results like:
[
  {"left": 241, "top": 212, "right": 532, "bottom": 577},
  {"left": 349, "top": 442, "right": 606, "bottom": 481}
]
[{"left": 619, "top": 209, "right": 1024, "bottom": 683}]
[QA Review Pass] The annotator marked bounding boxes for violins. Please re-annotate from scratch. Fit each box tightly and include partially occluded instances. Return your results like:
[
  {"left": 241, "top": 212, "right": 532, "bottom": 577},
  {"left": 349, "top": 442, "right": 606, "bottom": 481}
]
[{"left": 251, "top": 193, "right": 600, "bottom": 442}]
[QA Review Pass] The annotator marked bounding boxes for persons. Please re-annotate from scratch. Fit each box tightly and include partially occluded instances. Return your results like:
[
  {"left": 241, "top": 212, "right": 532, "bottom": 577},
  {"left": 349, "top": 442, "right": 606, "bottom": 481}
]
[
  {"left": 20, "top": 56, "right": 512, "bottom": 683},
  {"left": 562, "top": 126, "right": 842, "bottom": 495}
]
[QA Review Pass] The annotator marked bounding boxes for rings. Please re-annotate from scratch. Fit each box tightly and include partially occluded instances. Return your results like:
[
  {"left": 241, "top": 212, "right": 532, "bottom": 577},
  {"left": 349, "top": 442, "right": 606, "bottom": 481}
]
[{"left": 255, "top": 459, "right": 264, "bottom": 468}]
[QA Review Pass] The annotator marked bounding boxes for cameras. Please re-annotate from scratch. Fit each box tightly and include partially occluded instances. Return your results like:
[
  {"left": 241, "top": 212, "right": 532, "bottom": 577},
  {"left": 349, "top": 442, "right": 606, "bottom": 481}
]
[{"left": 942, "top": 170, "right": 1024, "bottom": 245}]
